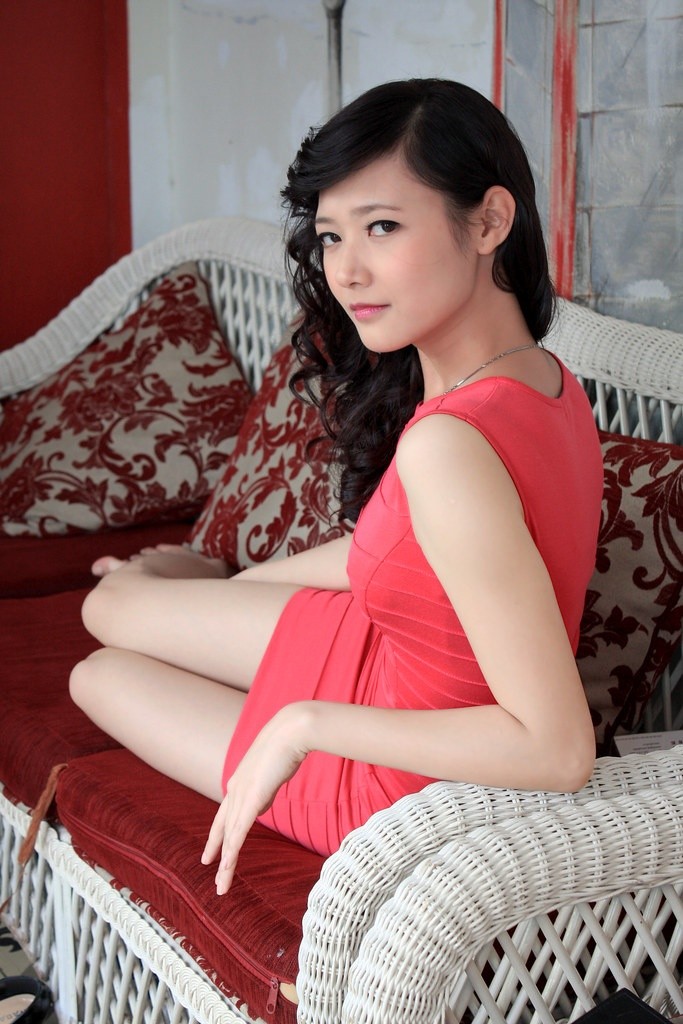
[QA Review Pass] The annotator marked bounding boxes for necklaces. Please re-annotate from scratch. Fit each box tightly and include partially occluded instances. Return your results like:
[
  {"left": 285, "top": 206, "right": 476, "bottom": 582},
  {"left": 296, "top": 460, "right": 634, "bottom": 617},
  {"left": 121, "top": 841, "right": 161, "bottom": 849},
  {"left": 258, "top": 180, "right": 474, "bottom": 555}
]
[{"left": 444, "top": 343, "right": 539, "bottom": 392}]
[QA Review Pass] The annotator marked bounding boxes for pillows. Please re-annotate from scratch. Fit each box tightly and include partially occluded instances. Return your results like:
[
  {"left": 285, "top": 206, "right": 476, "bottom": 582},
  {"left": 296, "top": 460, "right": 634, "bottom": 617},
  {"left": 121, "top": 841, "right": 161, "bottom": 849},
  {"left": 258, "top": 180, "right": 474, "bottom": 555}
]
[
  {"left": 574, "top": 428, "right": 683, "bottom": 754},
  {"left": 0, "top": 261, "right": 252, "bottom": 539},
  {"left": 185, "top": 310, "right": 357, "bottom": 569}
]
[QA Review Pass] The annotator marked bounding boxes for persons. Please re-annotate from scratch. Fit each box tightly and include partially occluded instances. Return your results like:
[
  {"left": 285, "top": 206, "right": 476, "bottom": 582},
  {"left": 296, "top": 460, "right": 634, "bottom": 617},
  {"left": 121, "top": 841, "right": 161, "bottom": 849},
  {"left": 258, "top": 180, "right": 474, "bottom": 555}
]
[{"left": 71, "top": 76, "right": 603, "bottom": 897}]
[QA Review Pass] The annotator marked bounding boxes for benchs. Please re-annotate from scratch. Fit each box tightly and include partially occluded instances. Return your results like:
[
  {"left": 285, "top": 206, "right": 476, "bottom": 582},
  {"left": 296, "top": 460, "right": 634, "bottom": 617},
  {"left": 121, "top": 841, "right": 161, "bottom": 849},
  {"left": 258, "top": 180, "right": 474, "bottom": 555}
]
[{"left": 1, "top": 218, "right": 683, "bottom": 1024}]
